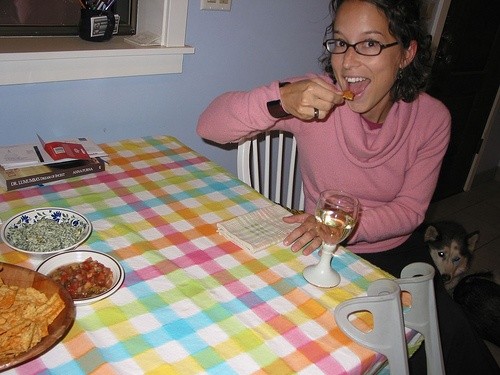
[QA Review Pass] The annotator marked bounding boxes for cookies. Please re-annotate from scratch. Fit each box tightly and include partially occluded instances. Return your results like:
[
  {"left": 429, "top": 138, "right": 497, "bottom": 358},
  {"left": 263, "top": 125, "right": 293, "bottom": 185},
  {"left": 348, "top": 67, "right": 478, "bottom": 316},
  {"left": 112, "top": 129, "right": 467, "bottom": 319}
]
[
  {"left": 0, "top": 279, "right": 65, "bottom": 365},
  {"left": 342, "top": 90, "right": 353, "bottom": 101}
]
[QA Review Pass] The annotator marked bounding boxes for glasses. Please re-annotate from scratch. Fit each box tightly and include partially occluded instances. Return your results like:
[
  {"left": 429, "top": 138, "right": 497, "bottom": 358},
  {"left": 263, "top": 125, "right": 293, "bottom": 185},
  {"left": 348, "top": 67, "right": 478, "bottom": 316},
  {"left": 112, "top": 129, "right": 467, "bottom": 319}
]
[{"left": 322, "top": 38, "right": 402, "bottom": 56}]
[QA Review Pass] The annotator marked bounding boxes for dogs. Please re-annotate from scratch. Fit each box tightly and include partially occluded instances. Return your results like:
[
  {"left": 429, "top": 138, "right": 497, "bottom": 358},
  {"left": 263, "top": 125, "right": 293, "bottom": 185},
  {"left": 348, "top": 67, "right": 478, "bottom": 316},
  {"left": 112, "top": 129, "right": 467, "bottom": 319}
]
[{"left": 412, "top": 220, "right": 479, "bottom": 302}]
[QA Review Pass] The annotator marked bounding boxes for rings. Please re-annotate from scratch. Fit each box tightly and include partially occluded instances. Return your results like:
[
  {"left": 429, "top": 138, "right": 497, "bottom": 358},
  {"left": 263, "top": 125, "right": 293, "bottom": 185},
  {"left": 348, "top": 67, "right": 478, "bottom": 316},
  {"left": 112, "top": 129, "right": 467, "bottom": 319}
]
[{"left": 314, "top": 108, "right": 319, "bottom": 121}]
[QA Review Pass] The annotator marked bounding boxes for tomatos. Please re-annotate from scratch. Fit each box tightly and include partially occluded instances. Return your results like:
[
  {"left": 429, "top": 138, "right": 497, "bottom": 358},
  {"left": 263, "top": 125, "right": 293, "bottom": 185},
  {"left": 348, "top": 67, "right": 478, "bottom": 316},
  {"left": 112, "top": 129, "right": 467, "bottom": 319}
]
[{"left": 57, "top": 257, "right": 112, "bottom": 297}]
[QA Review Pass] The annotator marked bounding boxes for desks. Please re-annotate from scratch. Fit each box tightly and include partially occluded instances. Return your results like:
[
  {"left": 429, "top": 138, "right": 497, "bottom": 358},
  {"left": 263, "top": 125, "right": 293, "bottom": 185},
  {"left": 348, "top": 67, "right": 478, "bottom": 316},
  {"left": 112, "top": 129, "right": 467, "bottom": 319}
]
[{"left": 236, "top": 130, "right": 305, "bottom": 211}]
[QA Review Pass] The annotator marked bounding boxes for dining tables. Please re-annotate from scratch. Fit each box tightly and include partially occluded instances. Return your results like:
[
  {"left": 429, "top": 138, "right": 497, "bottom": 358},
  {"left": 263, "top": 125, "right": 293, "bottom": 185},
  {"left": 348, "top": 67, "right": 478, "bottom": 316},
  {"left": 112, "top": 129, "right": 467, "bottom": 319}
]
[{"left": 0, "top": 136, "right": 424, "bottom": 375}]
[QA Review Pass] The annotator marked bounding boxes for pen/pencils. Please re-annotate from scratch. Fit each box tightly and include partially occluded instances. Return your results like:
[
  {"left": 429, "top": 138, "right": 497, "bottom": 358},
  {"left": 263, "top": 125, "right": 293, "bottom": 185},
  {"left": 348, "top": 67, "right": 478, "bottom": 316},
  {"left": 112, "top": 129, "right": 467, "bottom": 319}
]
[{"left": 78, "top": 0, "right": 119, "bottom": 12}]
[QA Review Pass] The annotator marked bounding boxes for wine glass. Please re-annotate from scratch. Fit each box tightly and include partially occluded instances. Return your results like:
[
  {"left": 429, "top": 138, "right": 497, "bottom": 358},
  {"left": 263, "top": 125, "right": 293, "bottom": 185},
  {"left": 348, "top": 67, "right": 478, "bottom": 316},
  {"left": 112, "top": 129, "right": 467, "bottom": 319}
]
[{"left": 302, "top": 189, "right": 360, "bottom": 288}]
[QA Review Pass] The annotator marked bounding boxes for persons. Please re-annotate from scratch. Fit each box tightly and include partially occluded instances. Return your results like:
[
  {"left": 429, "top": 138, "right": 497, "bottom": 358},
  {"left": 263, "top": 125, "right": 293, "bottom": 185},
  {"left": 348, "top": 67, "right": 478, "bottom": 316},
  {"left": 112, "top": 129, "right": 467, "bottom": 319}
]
[{"left": 197, "top": 0, "right": 452, "bottom": 307}]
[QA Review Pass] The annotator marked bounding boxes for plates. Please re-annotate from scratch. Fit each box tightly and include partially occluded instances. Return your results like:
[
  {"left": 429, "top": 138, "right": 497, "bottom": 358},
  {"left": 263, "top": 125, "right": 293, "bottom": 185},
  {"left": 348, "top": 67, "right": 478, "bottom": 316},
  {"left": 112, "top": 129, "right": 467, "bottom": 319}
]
[
  {"left": 1, "top": 207, "right": 92, "bottom": 255},
  {"left": 0, "top": 260, "right": 77, "bottom": 371},
  {"left": 35, "top": 249, "right": 125, "bottom": 306}
]
